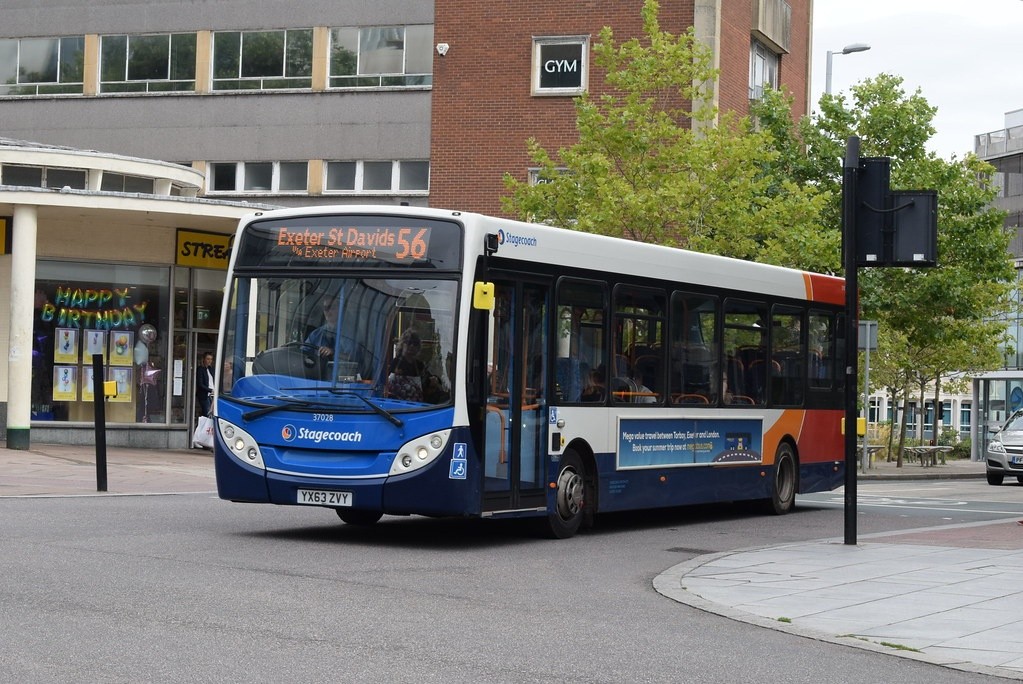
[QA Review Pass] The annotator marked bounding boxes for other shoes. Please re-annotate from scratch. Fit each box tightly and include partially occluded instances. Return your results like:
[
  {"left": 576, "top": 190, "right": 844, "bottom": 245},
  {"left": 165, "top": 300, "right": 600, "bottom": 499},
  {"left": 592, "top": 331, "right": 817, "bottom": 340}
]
[{"left": 194, "top": 443, "right": 203, "bottom": 448}]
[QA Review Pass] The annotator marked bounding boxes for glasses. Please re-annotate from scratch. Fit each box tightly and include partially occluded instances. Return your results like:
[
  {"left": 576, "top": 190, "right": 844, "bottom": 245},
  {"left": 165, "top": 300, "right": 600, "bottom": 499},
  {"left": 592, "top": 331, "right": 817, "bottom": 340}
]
[
  {"left": 403, "top": 340, "right": 420, "bottom": 346},
  {"left": 322, "top": 304, "right": 339, "bottom": 311}
]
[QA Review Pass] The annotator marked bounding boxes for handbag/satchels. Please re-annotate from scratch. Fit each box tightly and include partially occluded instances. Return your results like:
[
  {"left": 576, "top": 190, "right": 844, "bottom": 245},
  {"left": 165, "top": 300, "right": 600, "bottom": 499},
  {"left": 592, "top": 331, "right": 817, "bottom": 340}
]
[
  {"left": 192, "top": 415, "right": 214, "bottom": 448},
  {"left": 387, "top": 373, "right": 423, "bottom": 402}
]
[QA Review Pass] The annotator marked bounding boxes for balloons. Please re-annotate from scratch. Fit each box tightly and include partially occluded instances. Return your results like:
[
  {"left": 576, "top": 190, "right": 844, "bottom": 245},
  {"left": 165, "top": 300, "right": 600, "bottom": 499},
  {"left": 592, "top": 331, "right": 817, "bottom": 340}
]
[
  {"left": 138, "top": 324, "right": 158, "bottom": 343},
  {"left": 137, "top": 361, "right": 163, "bottom": 387},
  {"left": 134, "top": 340, "right": 150, "bottom": 364}
]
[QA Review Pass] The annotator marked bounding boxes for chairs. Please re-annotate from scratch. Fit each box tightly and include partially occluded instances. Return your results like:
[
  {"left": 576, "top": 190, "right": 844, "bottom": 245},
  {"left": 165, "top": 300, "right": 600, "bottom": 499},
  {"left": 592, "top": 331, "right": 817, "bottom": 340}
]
[{"left": 323, "top": 339, "right": 848, "bottom": 413}]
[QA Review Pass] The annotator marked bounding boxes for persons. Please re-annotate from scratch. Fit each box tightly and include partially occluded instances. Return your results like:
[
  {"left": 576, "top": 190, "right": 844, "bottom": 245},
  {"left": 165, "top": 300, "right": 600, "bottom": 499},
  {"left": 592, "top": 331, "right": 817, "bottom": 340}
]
[
  {"left": 626, "top": 369, "right": 660, "bottom": 403},
  {"left": 387, "top": 355, "right": 425, "bottom": 403},
  {"left": 395, "top": 329, "right": 440, "bottom": 405},
  {"left": 579, "top": 363, "right": 603, "bottom": 404},
  {"left": 297, "top": 294, "right": 364, "bottom": 380},
  {"left": 196, "top": 351, "right": 215, "bottom": 416}
]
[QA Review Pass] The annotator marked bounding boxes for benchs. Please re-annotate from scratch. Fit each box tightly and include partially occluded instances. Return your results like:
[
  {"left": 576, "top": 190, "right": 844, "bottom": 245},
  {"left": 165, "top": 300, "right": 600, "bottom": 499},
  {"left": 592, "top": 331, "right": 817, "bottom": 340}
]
[{"left": 856, "top": 445, "right": 954, "bottom": 469}]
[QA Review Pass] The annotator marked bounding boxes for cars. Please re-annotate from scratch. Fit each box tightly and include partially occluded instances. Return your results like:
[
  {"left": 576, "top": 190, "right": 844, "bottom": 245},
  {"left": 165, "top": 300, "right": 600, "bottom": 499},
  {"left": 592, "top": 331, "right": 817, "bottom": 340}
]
[{"left": 986, "top": 408, "right": 1023, "bottom": 486}]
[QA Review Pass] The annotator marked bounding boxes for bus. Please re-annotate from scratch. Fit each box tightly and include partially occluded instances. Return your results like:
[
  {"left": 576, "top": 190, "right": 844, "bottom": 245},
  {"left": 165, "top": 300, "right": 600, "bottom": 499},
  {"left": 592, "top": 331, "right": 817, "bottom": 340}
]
[{"left": 212, "top": 203, "right": 849, "bottom": 541}]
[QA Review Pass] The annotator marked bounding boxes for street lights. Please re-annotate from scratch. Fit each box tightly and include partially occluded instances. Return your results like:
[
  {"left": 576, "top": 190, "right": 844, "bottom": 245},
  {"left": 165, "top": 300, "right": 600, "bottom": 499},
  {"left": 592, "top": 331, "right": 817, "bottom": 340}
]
[{"left": 825, "top": 42, "right": 871, "bottom": 140}]
